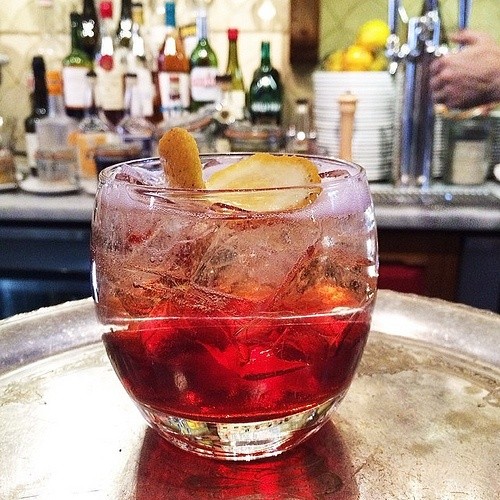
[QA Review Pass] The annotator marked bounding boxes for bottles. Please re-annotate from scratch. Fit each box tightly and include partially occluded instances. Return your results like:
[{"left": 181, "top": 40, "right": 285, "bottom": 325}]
[
  {"left": 24, "top": 55, "right": 51, "bottom": 176},
  {"left": 82, "top": 0, "right": 98, "bottom": 48},
  {"left": 36, "top": 70, "right": 79, "bottom": 185},
  {"left": 93, "top": 1, "right": 160, "bottom": 128},
  {"left": 189, "top": 15, "right": 218, "bottom": 114},
  {"left": 442, "top": 114, "right": 495, "bottom": 187},
  {"left": 156, "top": 0, "right": 191, "bottom": 120},
  {"left": 215, "top": 28, "right": 246, "bottom": 136},
  {"left": 246, "top": 41, "right": 283, "bottom": 126},
  {"left": 60, "top": 13, "right": 94, "bottom": 118},
  {"left": 285, "top": 97, "right": 317, "bottom": 156}
]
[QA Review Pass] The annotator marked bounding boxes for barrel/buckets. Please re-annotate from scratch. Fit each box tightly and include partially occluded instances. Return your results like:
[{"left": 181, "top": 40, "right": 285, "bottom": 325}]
[{"left": 311, "top": 69, "right": 403, "bottom": 181}]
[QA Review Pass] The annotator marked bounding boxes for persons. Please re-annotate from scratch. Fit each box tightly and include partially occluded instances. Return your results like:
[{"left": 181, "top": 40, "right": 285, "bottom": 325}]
[{"left": 428, "top": 29, "right": 500, "bottom": 108}]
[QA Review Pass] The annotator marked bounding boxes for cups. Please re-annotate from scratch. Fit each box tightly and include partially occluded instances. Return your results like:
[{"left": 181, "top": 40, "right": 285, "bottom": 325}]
[{"left": 92, "top": 152, "right": 378, "bottom": 463}]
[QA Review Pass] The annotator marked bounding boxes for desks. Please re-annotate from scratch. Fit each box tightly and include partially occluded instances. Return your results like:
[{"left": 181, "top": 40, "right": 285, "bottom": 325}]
[{"left": 0, "top": 180, "right": 500, "bottom": 320}]
